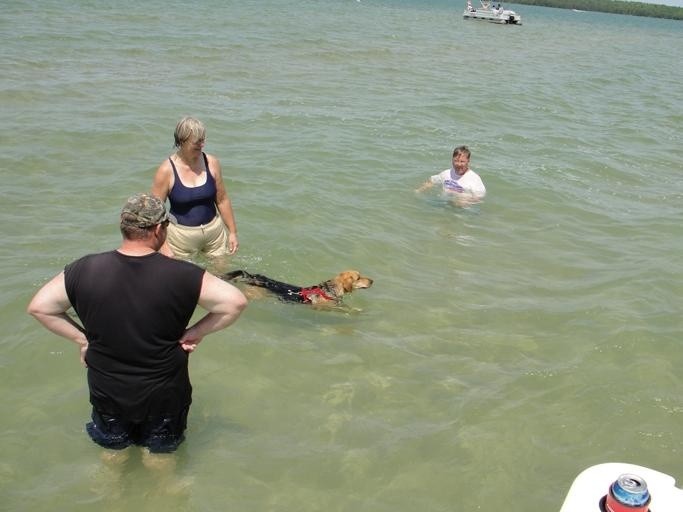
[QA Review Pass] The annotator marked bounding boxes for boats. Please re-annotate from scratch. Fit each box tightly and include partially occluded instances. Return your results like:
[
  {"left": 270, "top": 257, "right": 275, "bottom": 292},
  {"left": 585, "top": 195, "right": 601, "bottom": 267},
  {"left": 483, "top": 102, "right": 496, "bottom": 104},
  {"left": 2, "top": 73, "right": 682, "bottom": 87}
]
[{"left": 463, "top": 7, "right": 520, "bottom": 26}]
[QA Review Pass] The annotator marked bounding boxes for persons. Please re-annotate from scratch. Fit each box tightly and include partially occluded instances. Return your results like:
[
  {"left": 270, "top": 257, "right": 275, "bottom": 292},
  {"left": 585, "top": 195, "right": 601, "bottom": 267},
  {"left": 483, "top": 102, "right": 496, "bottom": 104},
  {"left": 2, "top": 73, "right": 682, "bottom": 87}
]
[
  {"left": 27, "top": 193, "right": 247, "bottom": 466},
  {"left": 415, "top": 146, "right": 486, "bottom": 208},
  {"left": 492, "top": 4, "right": 503, "bottom": 16},
  {"left": 151, "top": 118, "right": 238, "bottom": 265}
]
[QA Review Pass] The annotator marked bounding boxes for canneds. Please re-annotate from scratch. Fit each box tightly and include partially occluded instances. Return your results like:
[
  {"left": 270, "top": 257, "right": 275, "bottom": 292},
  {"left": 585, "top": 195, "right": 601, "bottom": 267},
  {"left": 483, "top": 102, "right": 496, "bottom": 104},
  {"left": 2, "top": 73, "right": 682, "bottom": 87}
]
[{"left": 604, "top": 473, "right": 651, "bottom": 512}]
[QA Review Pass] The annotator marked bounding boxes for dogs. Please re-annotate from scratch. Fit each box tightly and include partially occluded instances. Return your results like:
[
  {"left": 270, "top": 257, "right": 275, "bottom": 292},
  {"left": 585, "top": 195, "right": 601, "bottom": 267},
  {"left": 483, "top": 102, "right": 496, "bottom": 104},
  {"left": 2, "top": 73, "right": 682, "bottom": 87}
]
[{"left": 211, "top": 270, "right": 374, "bottom": 313}]
